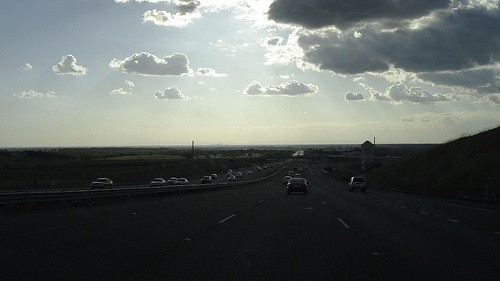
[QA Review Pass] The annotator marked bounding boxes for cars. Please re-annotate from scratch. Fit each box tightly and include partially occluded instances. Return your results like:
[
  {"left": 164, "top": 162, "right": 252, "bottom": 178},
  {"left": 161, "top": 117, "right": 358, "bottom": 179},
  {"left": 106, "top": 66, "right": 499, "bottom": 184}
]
[
  {"left": 289, "top": 158, "right": 329, "bottom": 176},
  {"left": 211, "top": 174, "right": 217, "bottom": 179},
  {"left": 178, "top": 178, "right": 188, "bottom": 185},
  {"left": 224, "top": 163, "right": 274, "bottom": 182},
  {"left": 283, "top": 176, "right": 291, "bottom": 182},
  {"left": 286, "top": 178, "right": 308, "bottom": 194},
  {"left": 288, "top": 171, "right": 294, "bottom": 176},
  {"left": 150, "top": 178, "right": 166, "bottom": 186},
  {"left": 90, "top": 178, "right": 114, "bottom": 189},
  {"left": 167, "top": 177, "right": 180, "bottom": 184},
  {"left": 201, "top": 176, "right": 212, "bottom": 183},
  {"left": 295, "top": 174, "right": 301, "bottom": 178}
]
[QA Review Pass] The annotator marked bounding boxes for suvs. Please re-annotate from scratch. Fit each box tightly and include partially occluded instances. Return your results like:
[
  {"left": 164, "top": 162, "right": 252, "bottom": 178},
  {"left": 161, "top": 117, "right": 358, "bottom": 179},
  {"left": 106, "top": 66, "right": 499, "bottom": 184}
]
[{"left": 349, "top": 177, "right": 367, "bottom": 192}]
